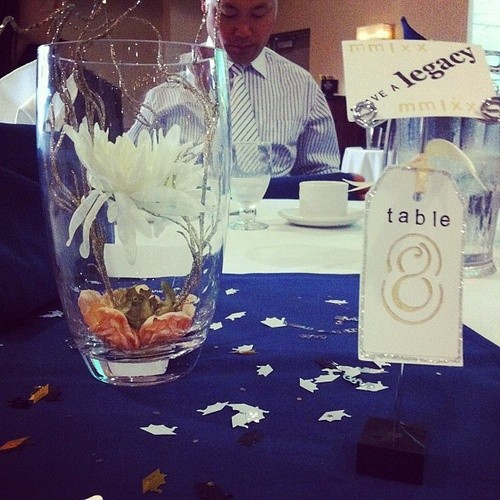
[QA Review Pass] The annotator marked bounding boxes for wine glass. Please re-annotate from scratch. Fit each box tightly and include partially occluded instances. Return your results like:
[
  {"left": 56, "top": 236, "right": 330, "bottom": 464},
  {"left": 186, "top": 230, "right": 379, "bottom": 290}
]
[{"left": 227, "top": 142, "right": 273, "bottom": 231}]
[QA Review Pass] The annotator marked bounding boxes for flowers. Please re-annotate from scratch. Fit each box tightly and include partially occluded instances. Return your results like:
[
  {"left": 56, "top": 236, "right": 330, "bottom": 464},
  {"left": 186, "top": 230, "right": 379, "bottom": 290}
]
[{"left": 62, "top": 118, "right": 207, "bottom": 264}]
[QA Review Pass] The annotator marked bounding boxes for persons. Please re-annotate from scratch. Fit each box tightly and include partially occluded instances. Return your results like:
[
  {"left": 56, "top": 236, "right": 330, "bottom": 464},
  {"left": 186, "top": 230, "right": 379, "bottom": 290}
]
[{"left": 125, "top": 0, "right": 369, "bottom": 200}]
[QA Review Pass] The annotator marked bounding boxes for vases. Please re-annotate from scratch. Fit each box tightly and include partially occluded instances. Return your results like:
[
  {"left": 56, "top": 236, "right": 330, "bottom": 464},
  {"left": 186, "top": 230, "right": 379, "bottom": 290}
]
[{"left": 35, "top": 40, "right": 231, "bottom": 387}]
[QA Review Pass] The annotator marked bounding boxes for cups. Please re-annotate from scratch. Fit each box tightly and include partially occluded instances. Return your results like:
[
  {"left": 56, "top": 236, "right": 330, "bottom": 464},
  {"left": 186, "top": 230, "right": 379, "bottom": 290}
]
[
  {"left": 299, "top": 180, "right": 348, "bottom": 221},
  {"left": 35, "top": 39, "right": 231, "bottom": 385},
  {"left": 390, "top": 50, "right": 499, "bottom": 279}
]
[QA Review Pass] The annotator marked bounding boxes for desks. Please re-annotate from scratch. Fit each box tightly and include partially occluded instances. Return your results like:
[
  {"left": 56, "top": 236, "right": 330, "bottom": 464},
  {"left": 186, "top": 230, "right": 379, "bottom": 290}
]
[
  {"left": 0, "top": 201, "right": 500, "bottom": 500},
  {"left": 341, "top": 147, "right": 398, "bottom": 185}
]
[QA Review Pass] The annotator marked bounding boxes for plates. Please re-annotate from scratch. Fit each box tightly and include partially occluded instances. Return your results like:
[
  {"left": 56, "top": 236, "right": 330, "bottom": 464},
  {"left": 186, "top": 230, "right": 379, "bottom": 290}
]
[{"left": 279, "top": 207, "right": 365, "bottom": 227}]
